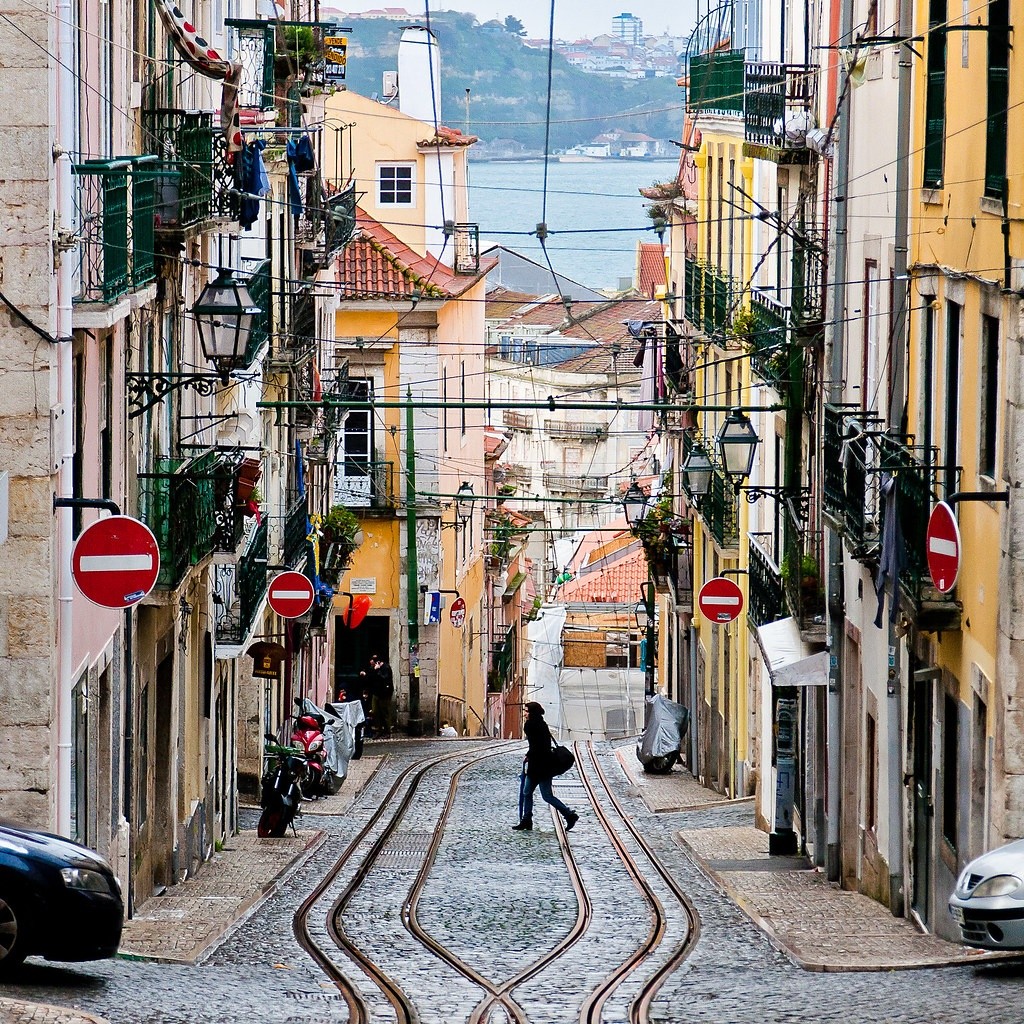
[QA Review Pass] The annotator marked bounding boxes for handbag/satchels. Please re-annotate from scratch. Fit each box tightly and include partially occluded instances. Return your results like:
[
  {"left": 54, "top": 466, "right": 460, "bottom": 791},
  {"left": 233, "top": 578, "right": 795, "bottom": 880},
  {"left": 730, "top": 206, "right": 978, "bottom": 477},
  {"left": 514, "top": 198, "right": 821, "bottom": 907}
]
[{"left": 551, "top": 745, "right": 575, "bottom": 777}]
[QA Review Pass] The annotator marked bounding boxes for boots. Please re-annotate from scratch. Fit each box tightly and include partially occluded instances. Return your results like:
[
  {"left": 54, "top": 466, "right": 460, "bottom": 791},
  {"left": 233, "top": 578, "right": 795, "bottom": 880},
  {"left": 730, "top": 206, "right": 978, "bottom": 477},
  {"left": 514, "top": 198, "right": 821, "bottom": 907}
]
[
  {"left": 512, "top": 817, "right": 532, "bottom": 830},
  {"left": 561, "top": 807, "right": 579, "bottom": 831}
]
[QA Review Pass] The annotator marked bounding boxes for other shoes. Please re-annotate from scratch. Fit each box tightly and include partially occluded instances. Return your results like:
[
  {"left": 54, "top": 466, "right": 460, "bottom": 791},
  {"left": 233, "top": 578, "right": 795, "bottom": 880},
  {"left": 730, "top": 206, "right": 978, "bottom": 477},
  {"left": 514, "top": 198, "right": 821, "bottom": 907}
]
[{"left": 371, "top": 728, "right": 391, "bottom": 739}]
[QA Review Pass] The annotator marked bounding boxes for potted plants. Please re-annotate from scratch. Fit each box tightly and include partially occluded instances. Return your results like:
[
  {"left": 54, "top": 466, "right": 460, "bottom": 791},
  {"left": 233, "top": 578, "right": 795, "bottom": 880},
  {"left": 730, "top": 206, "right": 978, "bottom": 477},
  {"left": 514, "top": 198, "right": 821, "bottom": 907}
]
[
  {"left": 491, "top": 546, "right": 501, "bottom": 567},
  {"left": 780, "top": 553, "right": 821, "bottom": 615}
]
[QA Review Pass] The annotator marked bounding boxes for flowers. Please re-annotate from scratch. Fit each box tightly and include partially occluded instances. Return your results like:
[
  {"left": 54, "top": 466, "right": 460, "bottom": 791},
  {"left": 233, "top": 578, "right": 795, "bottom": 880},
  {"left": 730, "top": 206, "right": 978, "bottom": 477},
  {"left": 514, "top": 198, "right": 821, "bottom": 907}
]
[
  {"left": 310, "top": 503, "right": 363, "bottom": 566},
  {"left": 635, "top": 497, "right": 690, "bottom": 566}
]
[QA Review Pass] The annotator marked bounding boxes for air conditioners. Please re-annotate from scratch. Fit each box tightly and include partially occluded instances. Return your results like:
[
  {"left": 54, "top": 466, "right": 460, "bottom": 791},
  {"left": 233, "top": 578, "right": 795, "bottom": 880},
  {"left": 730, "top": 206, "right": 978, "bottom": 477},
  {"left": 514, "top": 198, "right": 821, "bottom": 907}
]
[
  {"left": 455, "top": 230, "right": 472, "bottom": 267},
  {"left": 383, "top": 71, "right": 399, "bottom": 96}
]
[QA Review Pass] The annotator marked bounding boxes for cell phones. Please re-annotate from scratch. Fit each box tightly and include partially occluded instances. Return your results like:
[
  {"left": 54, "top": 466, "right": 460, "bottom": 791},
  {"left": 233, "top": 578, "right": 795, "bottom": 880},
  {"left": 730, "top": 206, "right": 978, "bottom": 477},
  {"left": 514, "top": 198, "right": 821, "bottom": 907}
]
[{"left": 376, "top": 661, "right": 381, "bottom": 665}]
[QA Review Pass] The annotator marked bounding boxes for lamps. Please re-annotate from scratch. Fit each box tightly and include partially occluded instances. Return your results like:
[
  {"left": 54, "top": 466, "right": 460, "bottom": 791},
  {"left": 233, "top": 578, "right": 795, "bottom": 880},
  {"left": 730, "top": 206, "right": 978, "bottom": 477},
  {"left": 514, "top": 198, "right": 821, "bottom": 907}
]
[
  {"left": 623, "top": 482, "right": 696, "bottom": 548},
  {"left": 124, "top": 268, "right": 262, "bottom": 419},
  {"left": 680, "top": 441, "right": 713, "bottom": 509},
  {"left": 715, "top": 407, "right": 812, "bottom": 523},
  {"left": 440, "top": 482, "right": 475, "bottom": 533},
  {"left": 635, "top": 601, "right": 659, "bottom": 636}
]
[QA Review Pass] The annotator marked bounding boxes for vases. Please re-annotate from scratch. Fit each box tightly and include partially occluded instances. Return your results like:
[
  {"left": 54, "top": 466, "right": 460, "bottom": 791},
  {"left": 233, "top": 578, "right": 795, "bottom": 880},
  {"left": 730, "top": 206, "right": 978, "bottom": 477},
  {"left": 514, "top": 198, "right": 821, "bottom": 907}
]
[
  {"left": 655, "top": 559, "right": 668, "bottom": 570},
  {"left": 237, "top": 458, "right": 262, "bottom": 499}
]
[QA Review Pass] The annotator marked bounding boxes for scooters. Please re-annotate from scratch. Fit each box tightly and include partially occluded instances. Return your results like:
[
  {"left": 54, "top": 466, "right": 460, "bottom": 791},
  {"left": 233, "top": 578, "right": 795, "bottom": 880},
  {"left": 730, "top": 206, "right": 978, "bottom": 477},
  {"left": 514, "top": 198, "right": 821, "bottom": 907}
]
[
  {"left": 256, "top": 695, "right": 366, "bottom": 838},
  {"left": 636, "top": 693, "right": 688, "bottom": 775}
]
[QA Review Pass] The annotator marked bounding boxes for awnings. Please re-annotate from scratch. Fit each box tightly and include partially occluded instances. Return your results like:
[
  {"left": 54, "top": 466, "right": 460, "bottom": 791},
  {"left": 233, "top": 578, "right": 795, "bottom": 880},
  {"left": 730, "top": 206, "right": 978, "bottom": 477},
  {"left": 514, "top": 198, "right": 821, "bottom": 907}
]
[{"left": 756, "top": 618, "right": 830, "bottom": 685}]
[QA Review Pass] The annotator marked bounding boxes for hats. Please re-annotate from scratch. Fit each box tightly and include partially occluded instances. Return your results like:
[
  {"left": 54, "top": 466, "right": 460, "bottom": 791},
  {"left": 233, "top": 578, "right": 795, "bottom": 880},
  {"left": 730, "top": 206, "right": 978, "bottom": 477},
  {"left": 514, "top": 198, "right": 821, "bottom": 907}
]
[{"left": 526, "top": 702, "right": 545, "bottom": 715}]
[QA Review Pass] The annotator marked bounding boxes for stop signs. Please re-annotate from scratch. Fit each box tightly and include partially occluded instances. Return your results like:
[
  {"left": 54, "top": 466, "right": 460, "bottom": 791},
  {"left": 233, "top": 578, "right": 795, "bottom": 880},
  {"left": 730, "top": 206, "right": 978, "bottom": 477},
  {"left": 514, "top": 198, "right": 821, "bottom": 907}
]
[
  {"left": 69, "top": 514, "right": 161, "bottom": 611},
  {"left": 266, "top": 570, "right": 314, "bottom": 619},
  {"left": 450, "top": 596, "right": 467, "bottom": 628},
  {"left": 924, "top": 501, "right": 962, "bottom": 593},
  {"left": 698, "top": 576, "right": 744, "bottom": 624}
]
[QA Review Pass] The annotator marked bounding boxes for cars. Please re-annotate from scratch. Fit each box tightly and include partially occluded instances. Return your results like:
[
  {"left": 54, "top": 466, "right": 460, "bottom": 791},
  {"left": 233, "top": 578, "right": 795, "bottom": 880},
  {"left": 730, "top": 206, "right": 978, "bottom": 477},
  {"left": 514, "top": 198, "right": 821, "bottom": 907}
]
[
  {"left": 947, "top": 838, "right": 1024, "bottom": 952},
  {"left": 0, "top": 813, "right": 126, "bottom": 976}
]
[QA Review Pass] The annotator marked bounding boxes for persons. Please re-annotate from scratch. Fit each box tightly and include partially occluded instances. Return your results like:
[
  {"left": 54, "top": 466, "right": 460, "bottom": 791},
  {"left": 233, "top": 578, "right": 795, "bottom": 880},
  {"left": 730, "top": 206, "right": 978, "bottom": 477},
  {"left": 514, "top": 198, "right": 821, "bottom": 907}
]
[
  {"left": 359, "top": 645, "right": 394, "bottom": 740},
  {"left": 512, "top": 702, "right": 579, "bottom": 831}
]
